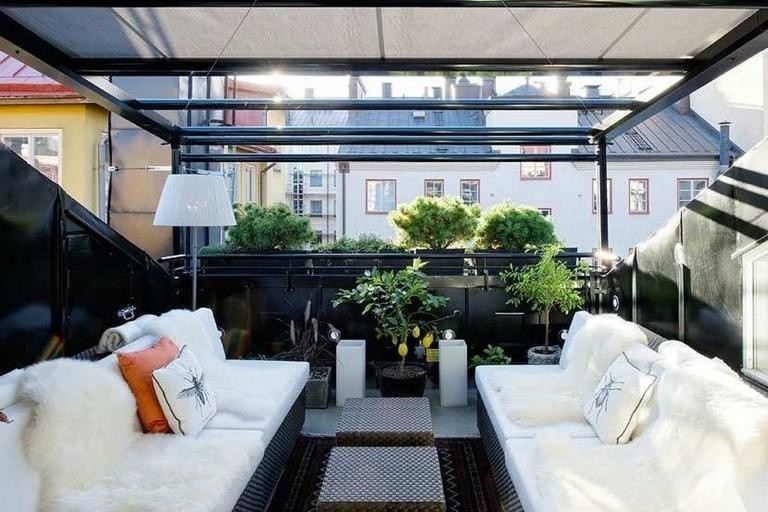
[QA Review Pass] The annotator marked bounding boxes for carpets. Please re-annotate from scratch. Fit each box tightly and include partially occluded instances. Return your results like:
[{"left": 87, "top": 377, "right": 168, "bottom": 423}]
[{"left": 264, "top": 435, "right": 505, "bottom": 512}]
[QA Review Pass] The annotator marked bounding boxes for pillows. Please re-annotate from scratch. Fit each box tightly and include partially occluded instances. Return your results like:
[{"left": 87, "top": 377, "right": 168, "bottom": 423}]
[
  {"left": 151, "top": 343, "right": 220, "bottom": 436},
  {"left": 580, "top": 351, "right": 657, "bottom": 447},
  {"left": 111, "top": 335, "right": 179, "bottom": 433}
]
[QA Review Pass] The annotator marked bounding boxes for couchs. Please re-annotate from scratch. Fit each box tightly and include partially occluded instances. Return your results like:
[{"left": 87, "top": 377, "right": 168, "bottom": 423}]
[
  {"left": 0, "top": 307, "right": 310, "bottom": 511},
  {"left": 472, "top": 310, "right": 767, "bottom": 509}
]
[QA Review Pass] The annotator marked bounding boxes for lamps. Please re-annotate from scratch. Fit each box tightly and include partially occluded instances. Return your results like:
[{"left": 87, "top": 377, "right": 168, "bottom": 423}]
[{"left": 152, "top": 174, "right": 237, "bottom": 308}]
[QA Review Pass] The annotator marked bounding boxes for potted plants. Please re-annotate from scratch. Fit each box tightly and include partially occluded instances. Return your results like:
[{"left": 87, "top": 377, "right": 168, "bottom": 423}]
[
  {"left": 261, "top": 288, "right": 341, "bottom": 409},
  {"left": 504, "top": 241, "right": 585, "bottom": 364},
  {"left": 329, "top": 256, "right": 454, "bottom": 397}
]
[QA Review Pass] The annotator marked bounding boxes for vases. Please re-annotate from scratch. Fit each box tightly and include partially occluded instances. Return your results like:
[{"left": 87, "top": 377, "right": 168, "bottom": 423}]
[
  {"left": 335, "top": 340, "right": 366, "bottom": 407},
  {"left": 439, "top": 339, "right": 468, "bottom": 407}
]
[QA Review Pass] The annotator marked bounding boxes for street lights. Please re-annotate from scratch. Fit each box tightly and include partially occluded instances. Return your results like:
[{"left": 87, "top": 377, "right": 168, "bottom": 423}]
[
  {"left": 338, "top": 161, "right": 350, "bottom": 241},
  {"left": 259, "top": 162, "right": 276, "bottom": 212}
]
